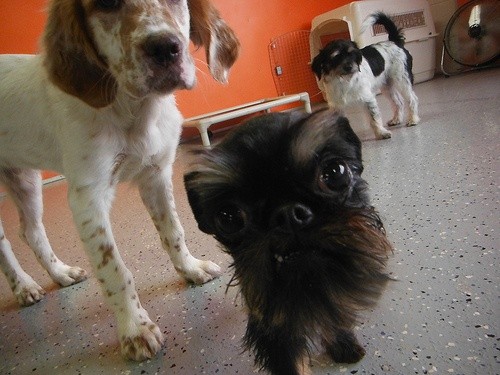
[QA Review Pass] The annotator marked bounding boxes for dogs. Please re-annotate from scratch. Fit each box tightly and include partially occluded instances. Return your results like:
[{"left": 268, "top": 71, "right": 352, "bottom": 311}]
[
  {"left": 0, "top": 0, "right": 240, "bottom": 362},
  {"left": 183, "top": 105, "right": 401, "bottom": 375},
  {"left": 311, "top": 10, "right": 421, "bottom": 140}
]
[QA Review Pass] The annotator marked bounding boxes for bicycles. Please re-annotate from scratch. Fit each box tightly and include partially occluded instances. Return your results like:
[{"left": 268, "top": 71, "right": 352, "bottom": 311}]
[{"left": 441, "top": 0, "right": 500, "bottom": 79}]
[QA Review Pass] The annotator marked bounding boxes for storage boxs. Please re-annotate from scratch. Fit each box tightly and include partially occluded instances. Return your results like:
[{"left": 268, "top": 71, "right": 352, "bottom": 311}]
[{"left": 309, "top": 0, "right": 440, "bottom": 101}]
